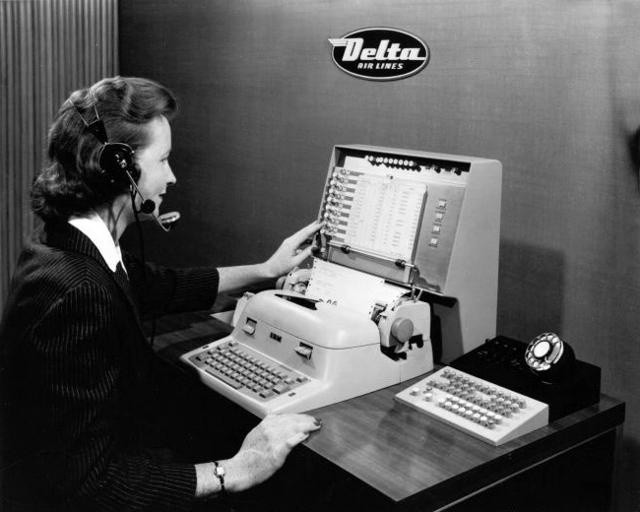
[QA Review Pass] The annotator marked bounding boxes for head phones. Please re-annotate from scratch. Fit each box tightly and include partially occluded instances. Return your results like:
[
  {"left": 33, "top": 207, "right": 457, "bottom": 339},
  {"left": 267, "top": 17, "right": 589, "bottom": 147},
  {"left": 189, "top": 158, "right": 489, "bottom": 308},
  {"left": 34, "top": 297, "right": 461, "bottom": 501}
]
[{"left": 67, "top": 88, "right": 141, "bottom": 191}]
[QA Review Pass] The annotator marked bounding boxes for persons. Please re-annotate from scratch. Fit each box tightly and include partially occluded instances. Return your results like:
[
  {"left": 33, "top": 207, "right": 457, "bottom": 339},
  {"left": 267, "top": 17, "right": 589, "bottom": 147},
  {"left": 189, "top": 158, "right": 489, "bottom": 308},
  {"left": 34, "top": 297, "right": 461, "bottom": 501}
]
[{"left": 0, "top": 77, "right": 327, "bottom": 512}]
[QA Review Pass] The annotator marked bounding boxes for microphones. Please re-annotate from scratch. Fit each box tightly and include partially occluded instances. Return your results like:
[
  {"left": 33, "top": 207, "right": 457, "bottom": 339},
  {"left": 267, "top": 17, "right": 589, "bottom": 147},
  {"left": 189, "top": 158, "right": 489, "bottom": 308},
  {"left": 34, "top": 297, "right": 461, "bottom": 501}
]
[{"left": 115, "top": 152, "right": 181, "bottom": 232}]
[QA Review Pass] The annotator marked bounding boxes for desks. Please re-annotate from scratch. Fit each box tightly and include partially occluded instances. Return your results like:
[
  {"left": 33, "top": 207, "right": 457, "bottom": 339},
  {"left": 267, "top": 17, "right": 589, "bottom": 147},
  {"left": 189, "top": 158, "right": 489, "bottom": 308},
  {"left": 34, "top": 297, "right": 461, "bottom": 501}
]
[{"left": 138, "top": 283, "right": 628, "bottom": 512}]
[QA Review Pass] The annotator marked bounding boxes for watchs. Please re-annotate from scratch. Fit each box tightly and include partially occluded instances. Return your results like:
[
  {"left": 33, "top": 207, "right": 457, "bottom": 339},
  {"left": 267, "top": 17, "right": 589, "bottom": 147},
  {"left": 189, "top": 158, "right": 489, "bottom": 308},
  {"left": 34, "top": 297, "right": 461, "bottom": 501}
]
[{"left": 214, "top": 461, "right": 226, "bottom": 491}]
